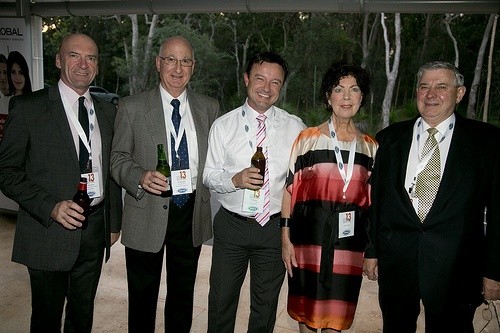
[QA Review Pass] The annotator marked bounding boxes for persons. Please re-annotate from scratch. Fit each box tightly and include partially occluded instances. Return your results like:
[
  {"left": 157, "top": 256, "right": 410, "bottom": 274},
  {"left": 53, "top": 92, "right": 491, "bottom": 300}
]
[
  {"left": 280, "top": 62, "right": 380, "bottom": 333},
  {"left": 110, "top": 36, "right": 221, "bottom": 333},
  {"left": 203, "top": 52, "right": 308, "bottom": 333},
  {"left": 362, "top": 61, "right": 500, "bottom": 333},
  {"left": 0, "top": 51, "right": 32, "bottom": 114},
  {"left": 0, "top": 32, "right": 123, "bottom": 333}
]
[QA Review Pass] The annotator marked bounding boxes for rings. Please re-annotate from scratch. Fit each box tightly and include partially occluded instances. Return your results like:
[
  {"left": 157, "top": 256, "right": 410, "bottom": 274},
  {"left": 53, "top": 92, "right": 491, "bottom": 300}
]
[
  {"left": 484, "top": 299, "right": 490, "bottom": 305},
  {"left": 148, "top": 182, "right": 152, "bottom": 188}
]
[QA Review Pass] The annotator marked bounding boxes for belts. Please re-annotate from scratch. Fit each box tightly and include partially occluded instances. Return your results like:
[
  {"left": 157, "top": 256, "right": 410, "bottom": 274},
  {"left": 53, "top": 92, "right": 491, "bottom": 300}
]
[
  {"left": 88, "top": 199, "right": 104, "bottom": 215},
  {"left": 223, "top": 207, "right": 282, "bottom": 223}
]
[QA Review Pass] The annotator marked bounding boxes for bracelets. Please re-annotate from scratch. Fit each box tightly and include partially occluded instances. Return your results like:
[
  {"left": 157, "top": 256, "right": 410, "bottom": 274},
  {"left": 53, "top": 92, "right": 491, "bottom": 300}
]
[{"left": 280, "top": 218, "right": 291, "bottom": 227}]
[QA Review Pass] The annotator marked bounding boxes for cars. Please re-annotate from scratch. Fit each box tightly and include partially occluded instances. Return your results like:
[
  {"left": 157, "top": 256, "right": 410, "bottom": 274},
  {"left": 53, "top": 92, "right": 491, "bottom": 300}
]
[{"left": 88, "top": 85, "right": 123, "bottom": 111}]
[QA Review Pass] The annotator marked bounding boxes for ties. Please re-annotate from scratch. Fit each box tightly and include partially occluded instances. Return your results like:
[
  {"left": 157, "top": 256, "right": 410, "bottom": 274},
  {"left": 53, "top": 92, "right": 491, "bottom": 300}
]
[
  {"left": 170, "top": 99, "right": 190, "bottom": 209},
  {"left": 78, "top": 97, "right": 95, "bottom": 206},
  {"left": 254, "top": 114, "right": 270, "bottom": 227},
  {"left": 414, "top": 128, "right": 441, "bottom": 222}
]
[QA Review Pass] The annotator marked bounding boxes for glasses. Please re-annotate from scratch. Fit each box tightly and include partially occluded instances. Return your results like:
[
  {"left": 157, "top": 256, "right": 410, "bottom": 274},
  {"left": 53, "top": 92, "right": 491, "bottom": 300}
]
[
  {"left": 158, "top": 55, "right": 194, "bottom": 67},
  {"left": 479, "top": 301, "right": 500, "bottom": 332}
]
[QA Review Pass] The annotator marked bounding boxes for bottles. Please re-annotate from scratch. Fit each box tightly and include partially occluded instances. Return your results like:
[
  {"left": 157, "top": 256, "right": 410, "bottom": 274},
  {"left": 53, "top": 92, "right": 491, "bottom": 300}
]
[
  {"left": 251, "top": 147, "right": 266, "bottom": 188},
  {"left": 73, "top": 177, "right": 89, "bottom": 230},
  {"left": 155, "top": 144, "right": 173, "bottom": 198}
]
